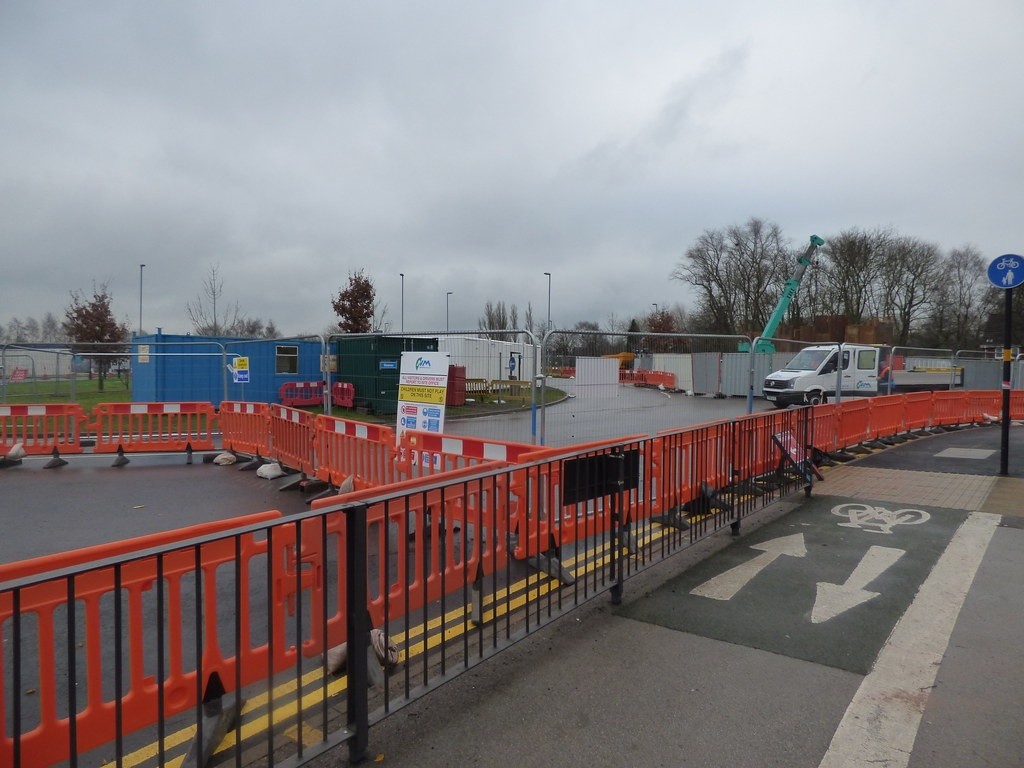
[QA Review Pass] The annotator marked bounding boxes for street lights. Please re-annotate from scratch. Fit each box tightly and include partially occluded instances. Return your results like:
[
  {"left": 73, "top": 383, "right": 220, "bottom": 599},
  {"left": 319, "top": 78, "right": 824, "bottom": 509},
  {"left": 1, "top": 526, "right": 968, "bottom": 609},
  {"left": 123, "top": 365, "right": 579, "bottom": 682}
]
[
  {"left": 400, "top": 273, "right": 404, "bottom": 333},
  {"left": 446, "top": 291, "right": 453, "bottom": 337},
  {"left": 139, "top": 264, "right": 146, "bottom": 336},
  {"left": 544, "top": 272, "right": 551, "bottom": 330}
]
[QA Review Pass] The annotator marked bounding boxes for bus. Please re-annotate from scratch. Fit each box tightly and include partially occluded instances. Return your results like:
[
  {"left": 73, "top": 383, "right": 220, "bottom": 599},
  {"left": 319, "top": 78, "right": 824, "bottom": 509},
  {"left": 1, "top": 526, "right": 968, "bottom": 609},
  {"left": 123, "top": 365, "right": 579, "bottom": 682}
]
[{"left": 544, "top": 353, "right": 575, "bottom": 379}]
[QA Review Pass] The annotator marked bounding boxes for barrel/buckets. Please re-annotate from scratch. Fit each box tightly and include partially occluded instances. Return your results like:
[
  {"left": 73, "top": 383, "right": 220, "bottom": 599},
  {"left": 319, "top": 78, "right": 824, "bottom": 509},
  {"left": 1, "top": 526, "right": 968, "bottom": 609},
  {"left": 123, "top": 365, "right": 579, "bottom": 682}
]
[{"left": 446, "top": 366, "right": 466, "bottom": 405}]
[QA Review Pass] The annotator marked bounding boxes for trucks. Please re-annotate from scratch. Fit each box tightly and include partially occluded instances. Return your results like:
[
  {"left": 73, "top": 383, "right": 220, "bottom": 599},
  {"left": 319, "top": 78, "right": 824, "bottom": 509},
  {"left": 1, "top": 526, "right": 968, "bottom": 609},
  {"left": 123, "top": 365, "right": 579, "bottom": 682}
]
[{"left": 761, "top": 343, "right": 965, "bottom": 409}]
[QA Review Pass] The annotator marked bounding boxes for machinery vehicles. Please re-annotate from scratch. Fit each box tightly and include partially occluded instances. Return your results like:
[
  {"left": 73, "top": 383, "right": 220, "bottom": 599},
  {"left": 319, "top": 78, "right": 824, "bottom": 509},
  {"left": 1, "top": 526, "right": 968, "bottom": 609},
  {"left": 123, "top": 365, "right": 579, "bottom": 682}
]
[{"left": 737, "top": 234, "right": 826, "bottom": 354}]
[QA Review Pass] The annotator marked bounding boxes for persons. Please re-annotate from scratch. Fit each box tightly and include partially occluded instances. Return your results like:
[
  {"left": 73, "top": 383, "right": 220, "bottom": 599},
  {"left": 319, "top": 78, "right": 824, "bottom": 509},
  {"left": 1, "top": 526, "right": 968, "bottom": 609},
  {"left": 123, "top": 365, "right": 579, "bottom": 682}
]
[
  {"left": 807, "top": 354, "right": 820, "bottom": 368},
  {"left": 876, "top": 361, "right": 894, "bottom": 396}
]
